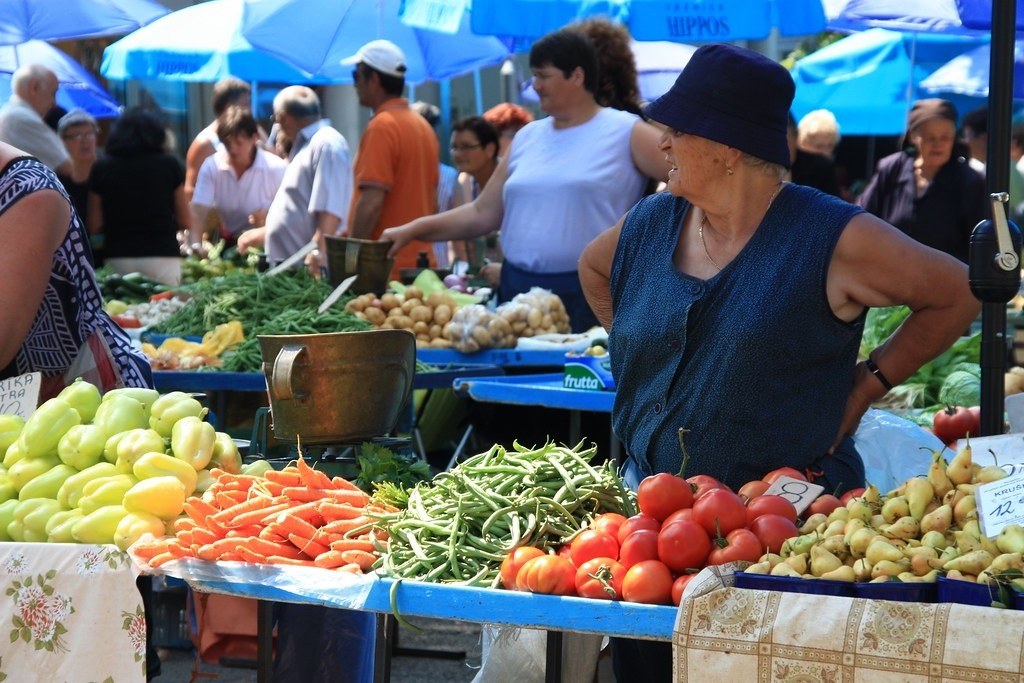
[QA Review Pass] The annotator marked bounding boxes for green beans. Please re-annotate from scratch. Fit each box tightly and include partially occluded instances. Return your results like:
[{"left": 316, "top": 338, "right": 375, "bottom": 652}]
[
  {"left": 346, "top": 435, "right": 646, "bottom": 633},
  {"left": 151, "top": 269, "right": 371, "bottom": 373}
]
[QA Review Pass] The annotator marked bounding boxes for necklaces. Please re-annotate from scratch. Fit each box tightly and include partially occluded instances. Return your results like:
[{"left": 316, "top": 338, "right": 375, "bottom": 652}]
[{"left": 699, "top": 183, "right": 784, "bottom": 271}]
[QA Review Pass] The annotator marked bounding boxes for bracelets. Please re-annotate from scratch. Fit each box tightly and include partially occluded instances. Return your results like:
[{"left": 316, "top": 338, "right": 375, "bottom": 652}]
[{"left": 865, "top": 359, "right": 893, "bottom": 392}]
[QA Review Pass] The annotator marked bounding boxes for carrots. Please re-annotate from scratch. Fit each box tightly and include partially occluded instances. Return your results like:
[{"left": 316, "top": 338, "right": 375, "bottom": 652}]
[{"left": 132, "top": 434, "right": 395, "bottom": 574}]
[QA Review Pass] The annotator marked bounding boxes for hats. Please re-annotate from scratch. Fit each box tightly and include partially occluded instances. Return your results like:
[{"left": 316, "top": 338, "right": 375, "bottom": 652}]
[
  {"left": 340, "top": 39, "right": 407, "bottom": 78},
  {"left": 646, "top": 44, "right": 796, "bottom": 172},
  {"left": 57, "top": 107, "right": 101, "bottom": 138}
]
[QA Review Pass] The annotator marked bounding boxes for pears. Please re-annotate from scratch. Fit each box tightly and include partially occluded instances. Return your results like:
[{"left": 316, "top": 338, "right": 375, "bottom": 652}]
[{"left": 740, "top": 433, "right": 1024, "bottom": 590}]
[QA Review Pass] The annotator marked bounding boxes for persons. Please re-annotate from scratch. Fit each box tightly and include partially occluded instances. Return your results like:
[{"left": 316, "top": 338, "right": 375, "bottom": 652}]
[
  {"left": 785, "top": 99, "right": 1024, "bottom": 266},
  {"left": 579, "top": 43, "right": 982, "bottom": 683},
  {"left": 378, "top": 32, "right": 672, "bottom": 468},
  {"left": 0, "top": 41, "right": 660, "bottom": 292},
  {"left": 0, "top": 140, "right": 163, "bottom": 683}
]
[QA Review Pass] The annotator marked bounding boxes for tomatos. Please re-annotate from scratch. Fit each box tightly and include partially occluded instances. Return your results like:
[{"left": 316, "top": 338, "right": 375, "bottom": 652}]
[
  {"left": 497, "top": 467, "right": 867, "bottom": 606},
  {"left": 922, "top": 403, "right": 979, "bottom": 451},
  {"left": 111, "top": 316, "right": 142, "bottom": 328}
]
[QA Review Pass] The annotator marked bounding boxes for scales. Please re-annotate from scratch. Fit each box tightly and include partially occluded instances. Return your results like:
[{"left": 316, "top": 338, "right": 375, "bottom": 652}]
[{"left": 249, "top": 407, "right": 416, "bottom": 479}]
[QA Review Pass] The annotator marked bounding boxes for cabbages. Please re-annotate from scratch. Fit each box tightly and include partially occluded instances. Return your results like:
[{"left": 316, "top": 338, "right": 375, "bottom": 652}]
[{"left": 859, "top": 302, "right": 983, "bottom": 405}]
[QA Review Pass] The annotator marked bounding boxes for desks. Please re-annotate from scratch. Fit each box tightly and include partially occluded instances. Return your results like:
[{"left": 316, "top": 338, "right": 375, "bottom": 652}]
[{"left": 0, "top": 333, "right": 1024, "bottom": 683}]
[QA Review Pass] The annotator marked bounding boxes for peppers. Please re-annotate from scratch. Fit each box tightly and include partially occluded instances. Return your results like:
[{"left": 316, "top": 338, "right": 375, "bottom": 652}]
[{"left": 0, "top": 376, "right": 272, "bottom": 554}]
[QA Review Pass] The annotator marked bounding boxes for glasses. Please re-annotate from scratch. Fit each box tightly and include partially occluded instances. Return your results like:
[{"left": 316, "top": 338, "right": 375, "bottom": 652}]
[
  {"left": 270, "top": 114, "right": 276, "bottom": 122},
  {"left": 63, "top": 131, "right": 96, "bottom": 143},
  {"left": 447, "top": 141, "right": 480, "bottom": 151},
  {"left": 351, "top": 70, "right": 359, "bottom": 80}
]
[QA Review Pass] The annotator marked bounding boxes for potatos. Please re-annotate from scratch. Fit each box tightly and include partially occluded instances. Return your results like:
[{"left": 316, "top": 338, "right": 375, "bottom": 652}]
[{"left": 344, "top": 279, "right": 571, "bottom": 352}]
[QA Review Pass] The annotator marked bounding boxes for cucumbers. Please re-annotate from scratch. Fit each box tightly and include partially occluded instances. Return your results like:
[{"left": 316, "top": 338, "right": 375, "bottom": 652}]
[{"left": 97, "top": 274, "right": 173, "bottom": 303}]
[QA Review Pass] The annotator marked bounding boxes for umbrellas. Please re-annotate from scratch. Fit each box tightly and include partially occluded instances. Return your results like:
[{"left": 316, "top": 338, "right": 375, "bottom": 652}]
[{"left": 0, "top": 0, "right": 1024, "bottom": 135}]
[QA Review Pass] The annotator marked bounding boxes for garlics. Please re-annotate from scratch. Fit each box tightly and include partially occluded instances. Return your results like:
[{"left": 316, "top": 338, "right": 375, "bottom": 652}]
[{"left": 121, "top": 299, "right": 176, "bottom": 325}]
